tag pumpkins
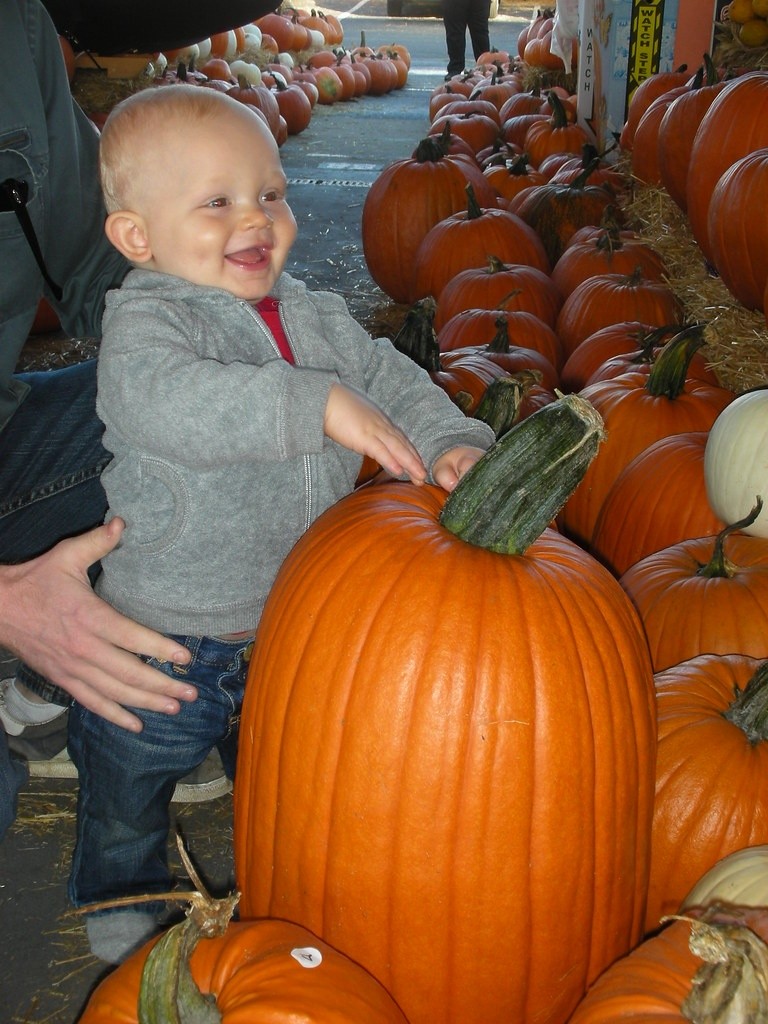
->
[53,834,410,1024]
[55,6,412,147]
[563,907,768,1024]
[229,386,655,1024]
[361,0,768,935]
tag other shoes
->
[444,73,459,81]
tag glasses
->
[1,178,65,302]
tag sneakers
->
[0,678,233,804]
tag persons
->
[63,84,500,968]
[442,0,493,81]
[0,0,234,846]
